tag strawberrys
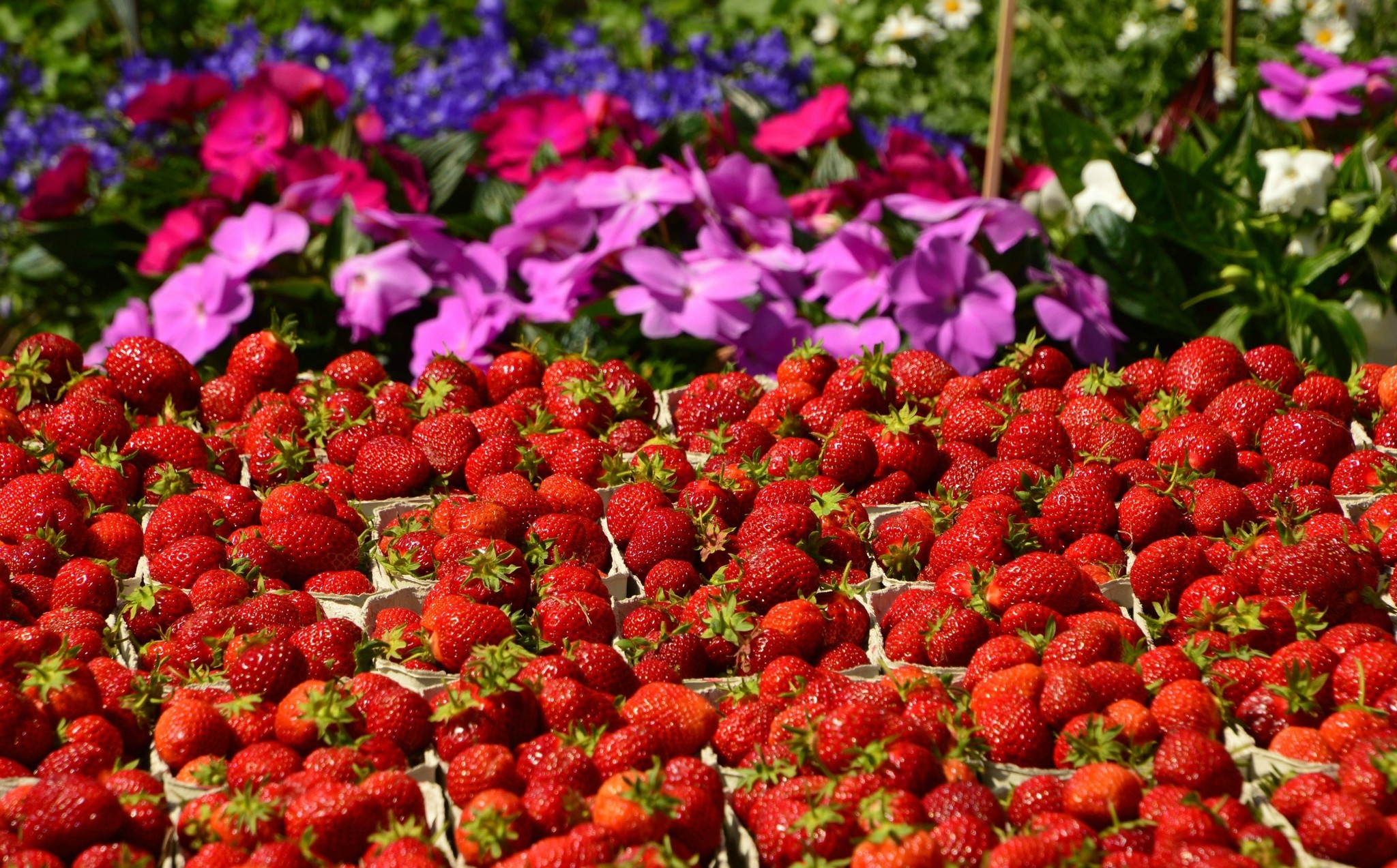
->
[0,311,1396,868]
